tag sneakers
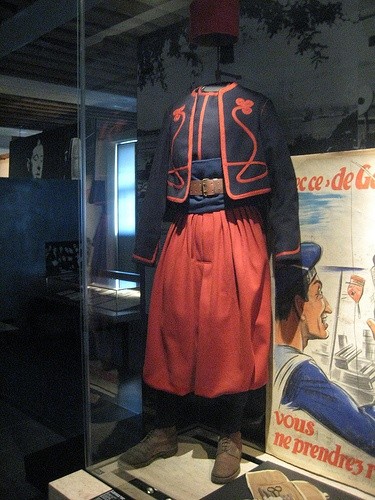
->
[211,430,242,484]
[118,427,178,470]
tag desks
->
[28,291,144,402]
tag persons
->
[117,81,301,484]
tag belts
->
[189,178,224,197]
